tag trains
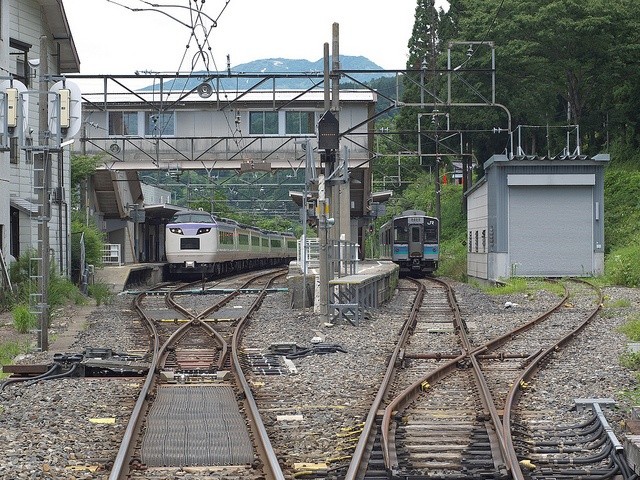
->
[376,209,440,277]
[163,207,297,282]
